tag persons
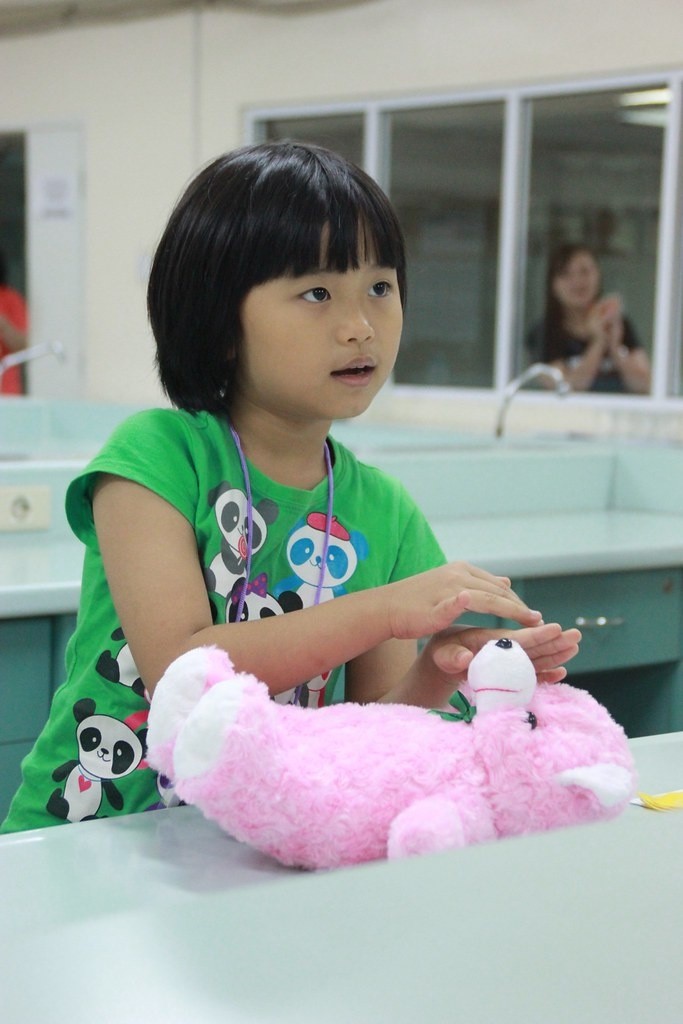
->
[525,244,651,399]
[0,145,580,837]
[0,286,29,397]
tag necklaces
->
[228,423,333,625]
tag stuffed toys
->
[144,636,637,873]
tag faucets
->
[488,362,571,434]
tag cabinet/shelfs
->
[0,509,683,832]
